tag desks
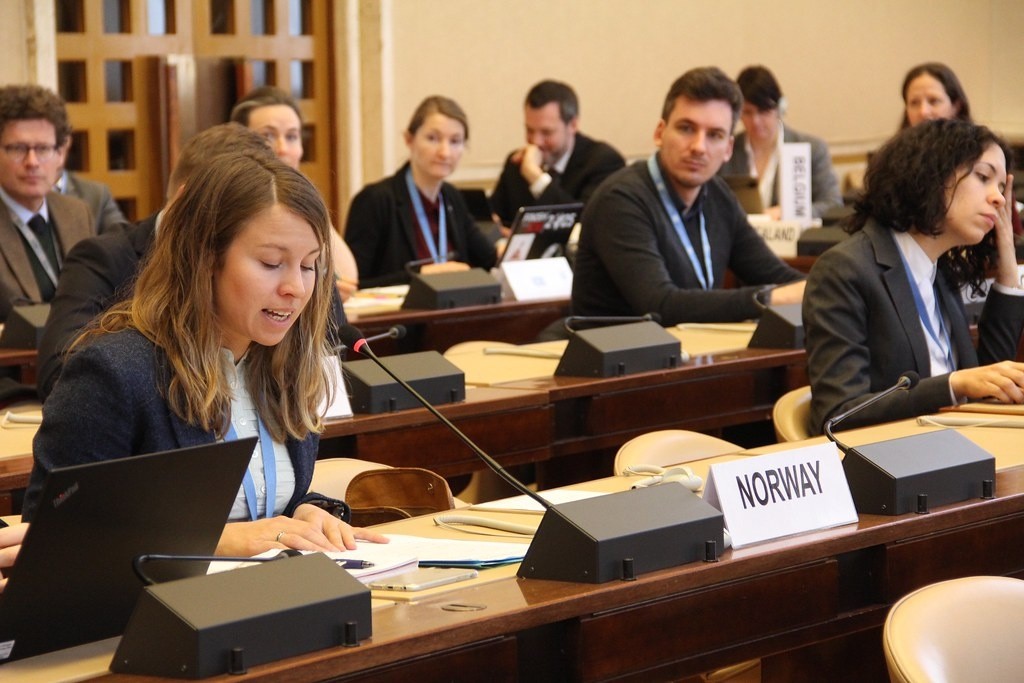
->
[0,260,1024,515]
[0,400,1024,683]
[0,252,823,388]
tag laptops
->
[496,202,587,269]
[0,436,259,664]
[719,172,764,214]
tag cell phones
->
[368,568,478,593]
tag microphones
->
[823,371,920,454]
[563,312,662,337]
[404,251,459,276]
[332,325,407,351]
[132,550,303,587]
[338,324,555,510]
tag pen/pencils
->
[332,559,374,569]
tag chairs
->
[305,339,1024,682]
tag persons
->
[892,60,1024,255]
[22,150,389,556]
[35,121,350,403]
[0,85,129,340]
[344,95,498,289]
[229,85,358,303]
[572,65,811,329]
[801,120,1024,437]
[491,80,628,258]
[720,67,843,223]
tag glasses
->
[0,142,61,161]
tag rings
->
[276,532,284,542]
